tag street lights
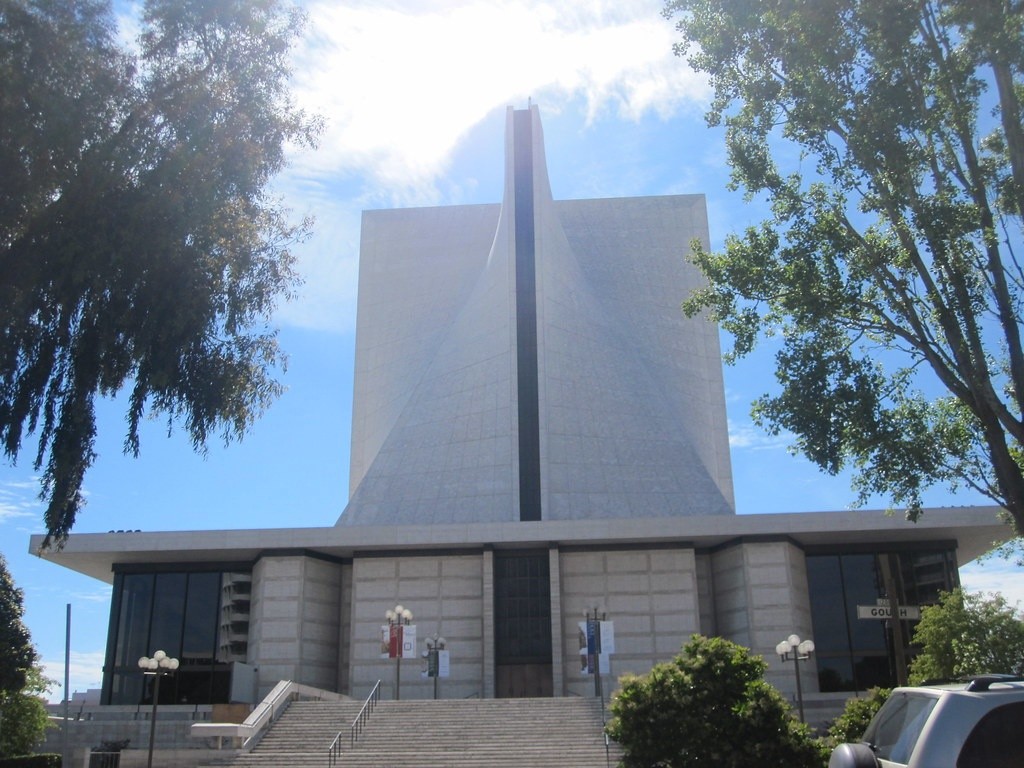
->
[775,633,814,724]
[581,599,611,698]
[138,650,180,768]
[385,605,414,700]
[425,634,447,700]
